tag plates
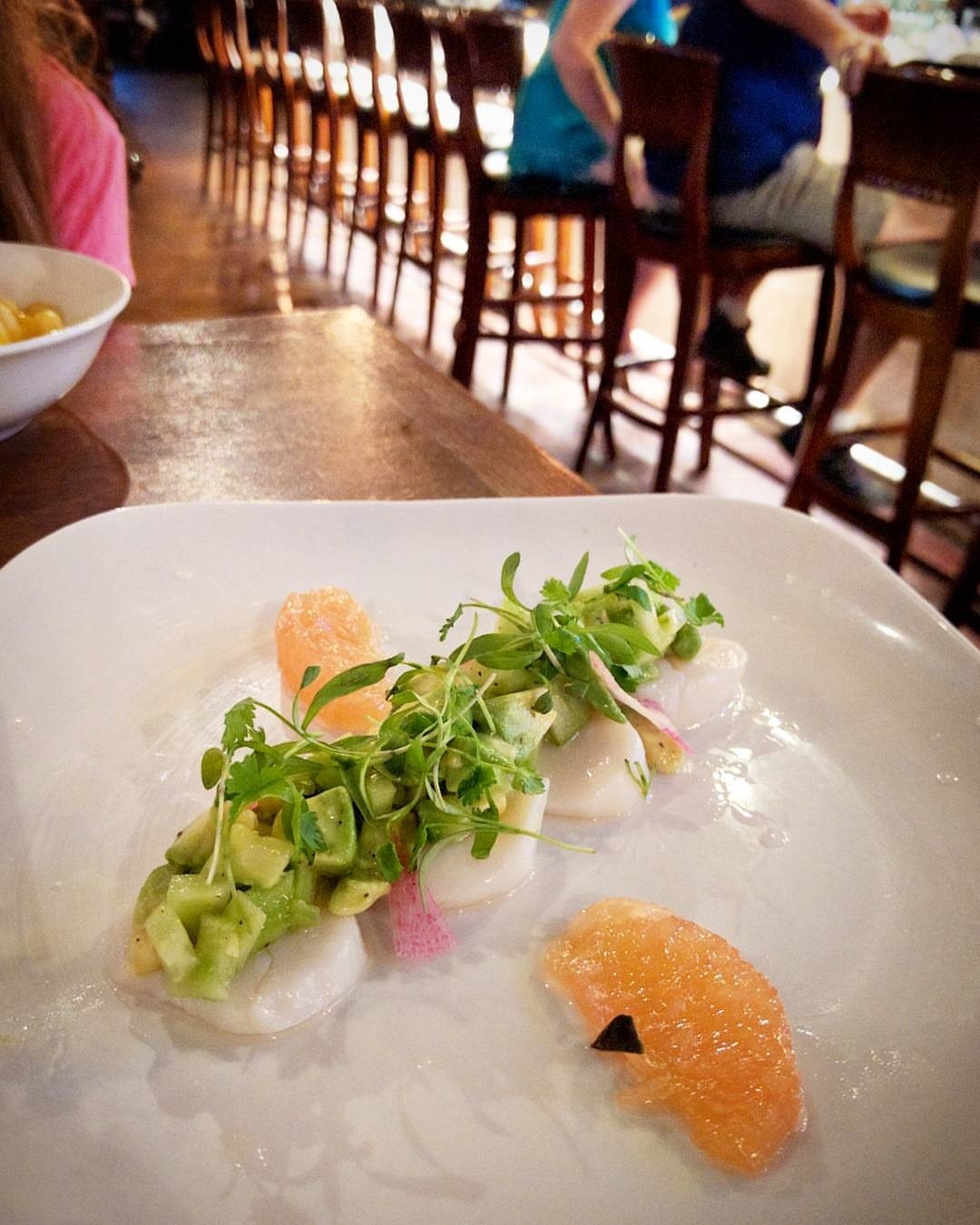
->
[0,492,980,1225]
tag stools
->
[200,0,980,637]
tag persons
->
[0,0,135,294]
[645,0,940,507]
[508,0,678,369]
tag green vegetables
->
[201,549,730,884]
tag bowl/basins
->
[1,240,133,443]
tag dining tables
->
[0,305,598,566]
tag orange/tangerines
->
[553,903,805,1174]
[277,587,394,734]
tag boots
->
[707,316,772,378]
